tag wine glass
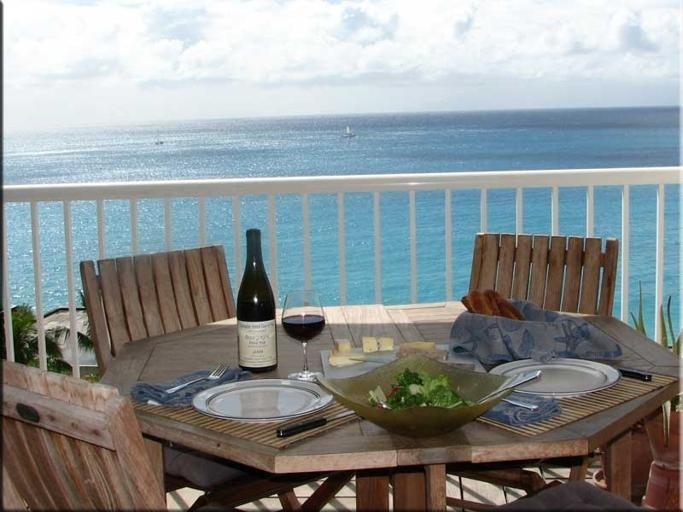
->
[281,289,325,382]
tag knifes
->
[277,408,356,438]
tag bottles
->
[236,229,278,374]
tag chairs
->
[1,235,680,512]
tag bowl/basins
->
[317,351,524,437]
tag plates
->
[486,358,621,398]
[191,379,334,420]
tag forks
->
[165,364,227,394]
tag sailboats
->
[342,125,357,139]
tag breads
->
[330,337,440,365]
[459,288,523,321]
